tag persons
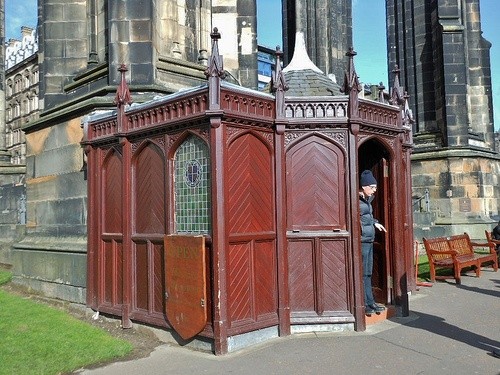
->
[356,170,388,314]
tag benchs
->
[422,232,498,287]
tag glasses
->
[369,185,377,190]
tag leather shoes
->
[365,305,374,314]
[370,303,385,312]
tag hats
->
[361,170,377,186]
